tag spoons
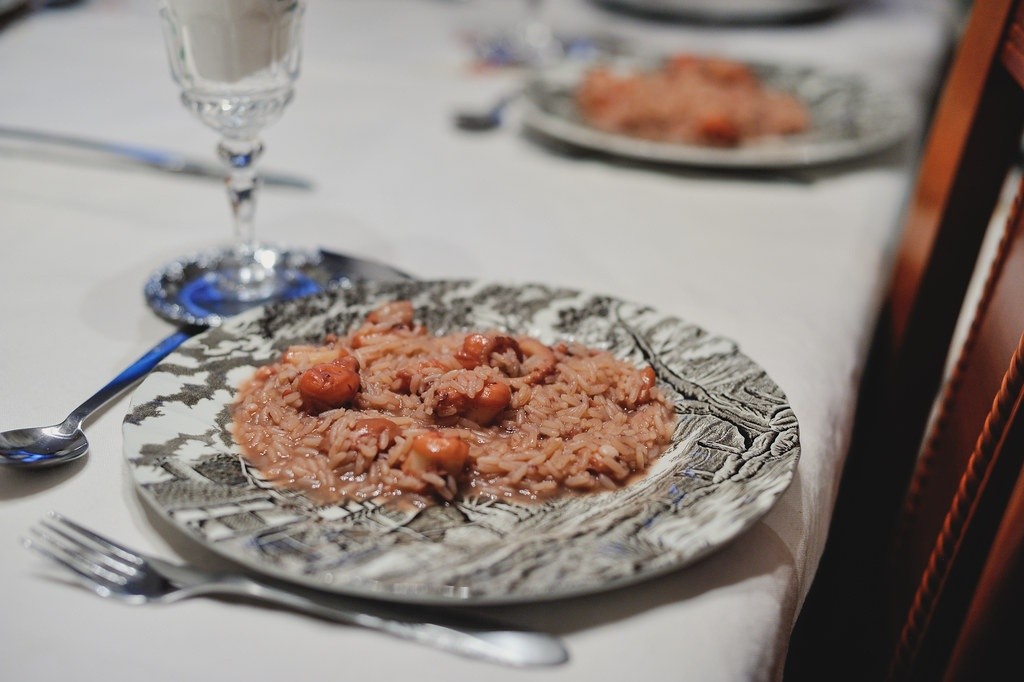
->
[0,323,206,470]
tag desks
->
[0,0,968,682]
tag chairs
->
[872,0,1024,682]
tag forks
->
[22,513,568,665]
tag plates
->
[522,41,924,166]
[122,278,801,605]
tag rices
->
[231,313,673,508]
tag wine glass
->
[145,0,368,325]
